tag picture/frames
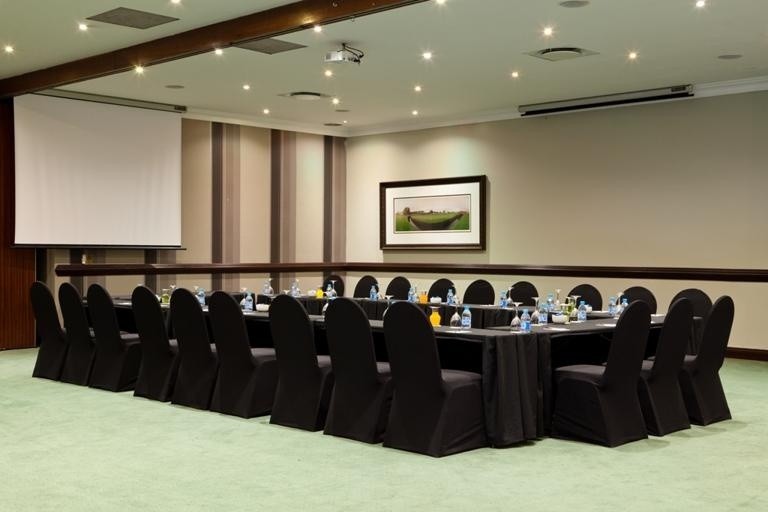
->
[379,174,486,252]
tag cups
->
[161,289,170,305]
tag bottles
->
[238,286,253,312]
[323,283,337,299]
[607,291,626,317]
[500,287,514,307]
[428,306,442,327]
[537,304,548,325]
[407,284,419,303]
[509,301,522,327]
[461,304,471,330]
[290,283,302,297]
[369,284,379,301]
[520,309,532,334]
[419,290,429,304]
[262,283,274,297]
[546,287,593,325]
[447,284,461,305]
[193,283,206,307]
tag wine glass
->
[531,296,539,325]
[382,293,394,319]
[448,301,461,330]
